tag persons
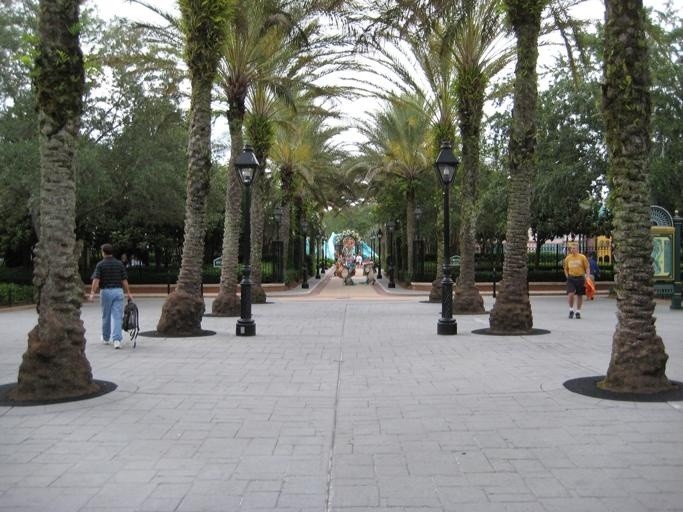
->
[89,242,134,350]
[562,242,590,320]
[585,251,600,301]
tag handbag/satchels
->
[123,298,139,348]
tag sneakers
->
[114,340,121,349]
[102,336,110,346]
[569,312,574,318]
[576,313,580,318]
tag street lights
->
[435,137,459,335]
[386,221,395,288]
[368,230,376,265]
[413,206,421,275]
[301,220,308,289]
[232,142,260,337]
[377,228,382,279]
[316,229,327,280]
[271,205,281,284]
